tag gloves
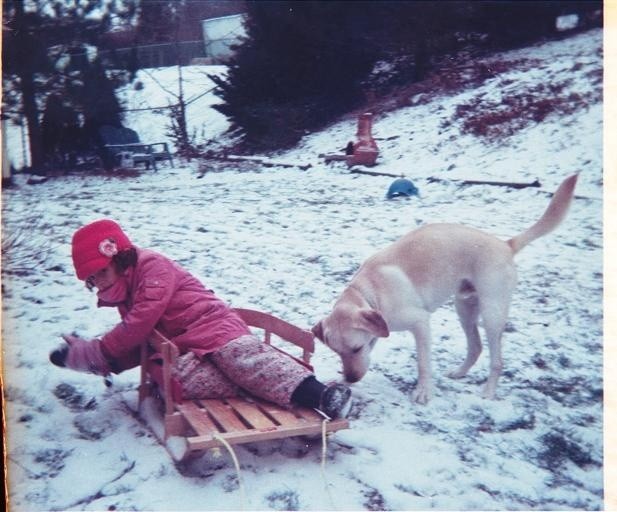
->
[51,331,112,381]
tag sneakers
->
[320,383,352,420]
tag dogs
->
[311,173,578,406]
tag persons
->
[50,219,352,422]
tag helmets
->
[387,179,418,199]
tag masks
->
[97,277,128,303]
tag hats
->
[72,219,132,279]
